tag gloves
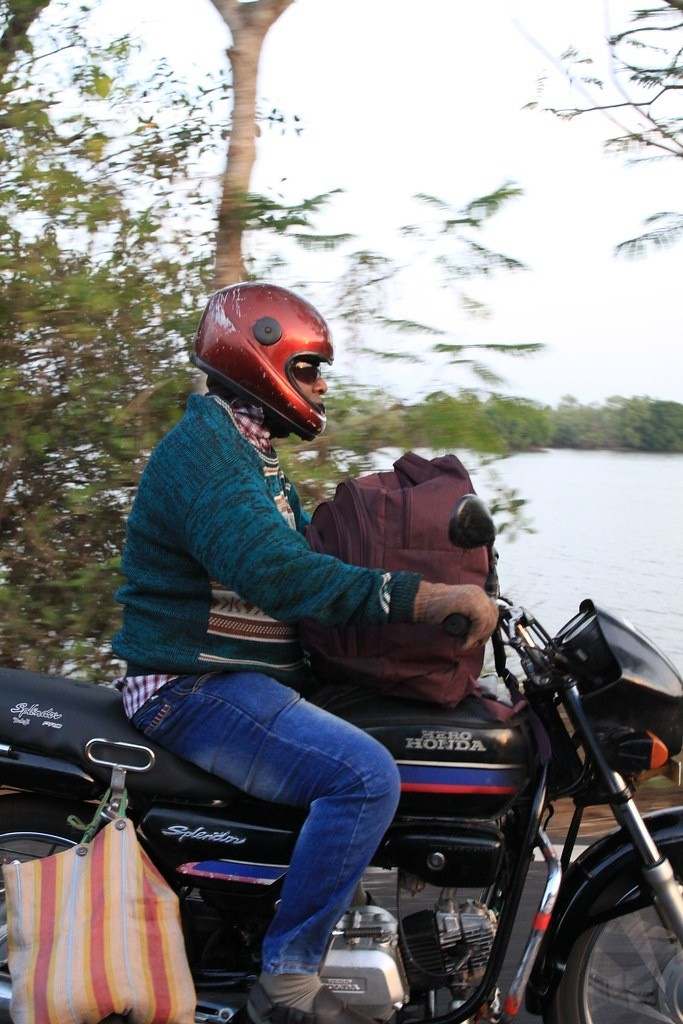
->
[413,580,500,650]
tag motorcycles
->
[1,493,683,1024]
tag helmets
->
[189,282,334,441]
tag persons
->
[114,284,498,1024]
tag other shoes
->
[247,980,380,1024]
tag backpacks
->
[304,450,552,764]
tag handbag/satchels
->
[2,788,197,1024]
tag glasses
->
[291,360,321,384]
